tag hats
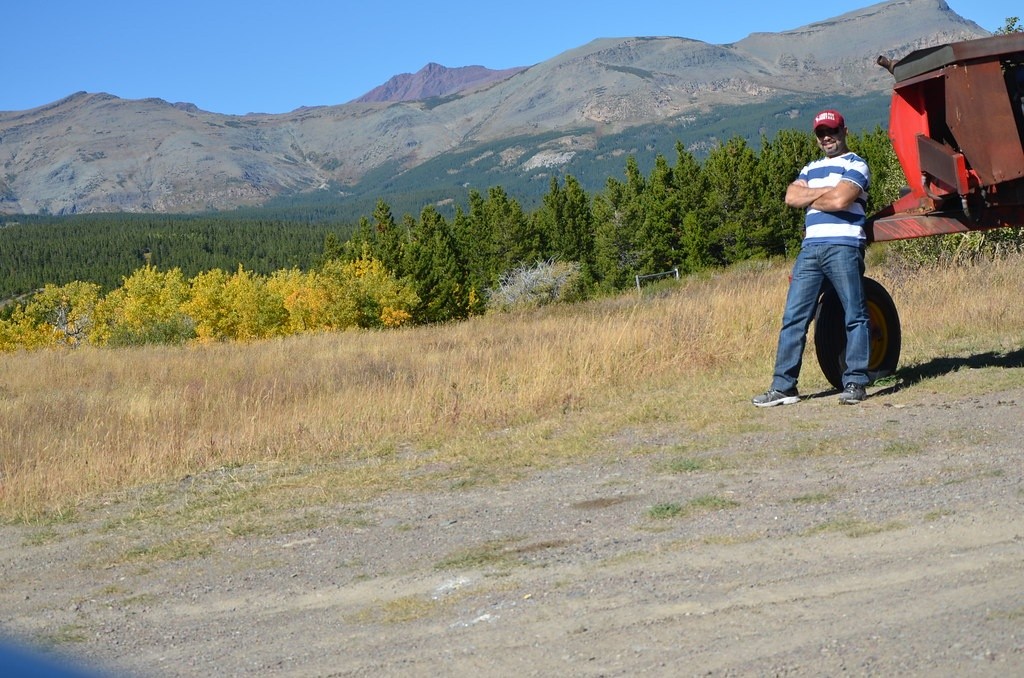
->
[813,111,844,132]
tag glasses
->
[816,128,839,137]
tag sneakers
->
[839,383,867,404]
[752,387,801,407]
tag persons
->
[750,109,873,408]
